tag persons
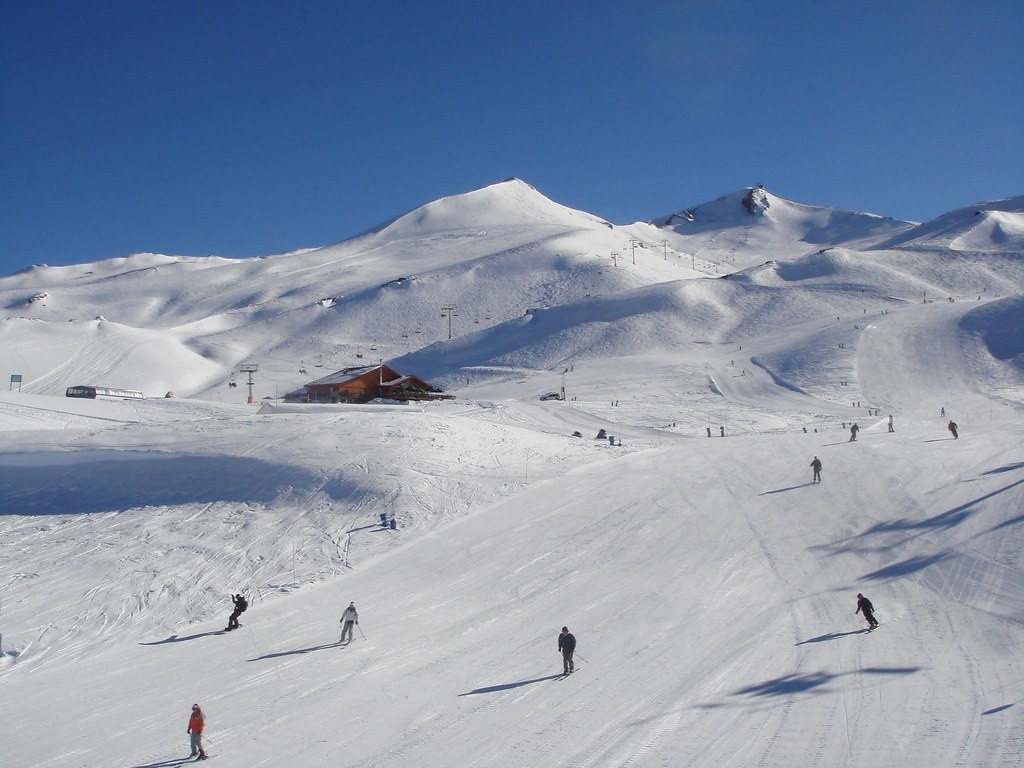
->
[187,704,206,759]
[855,593,879,629]
[558,626,577,673]
[225,594,244,631]
[850,423,860,442]
[810,456,822,484]
[948,420,958,439]
[338,601,359,643]
[888,415,895,432]
[940,407,946,418]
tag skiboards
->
[333,637,357,650]
[860,624,883,632]
[553,667,580,682]
[167,752,219,768]
[813,480,820,486]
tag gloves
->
[187,731,190,734]
[340,620,342,622]
[232,595,234,598]
[356,621,358,625]
[559,649,561,652]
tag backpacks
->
[240,601,248,611]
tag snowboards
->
[220,623,243,634]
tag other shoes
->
[190,752,198,756]
[564,670,568,674]
[570,669,573,673]
[232,625,238,628]
[870,624,874,629]
[875,624,879,628]
[225,628,231,630]
[348,640,351,643]
[198,753,206,758]
[340,639,344,642]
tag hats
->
[236,594,240,598]
[562,626,568,631]
[351,602,354,605]
[192,703,199,709]
[857,593,863,598]
